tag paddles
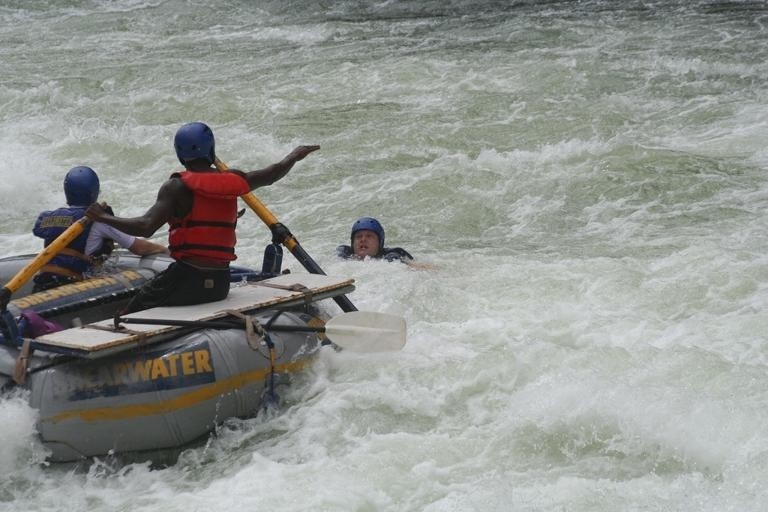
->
[111,309,407,355]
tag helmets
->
[62,165,99,205]
[175,122,216,169]
[350,217,384,257]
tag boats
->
[0,252,334,472]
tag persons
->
[338,217,414,262]
[84,122,321,314]
[32,165,171,292]
